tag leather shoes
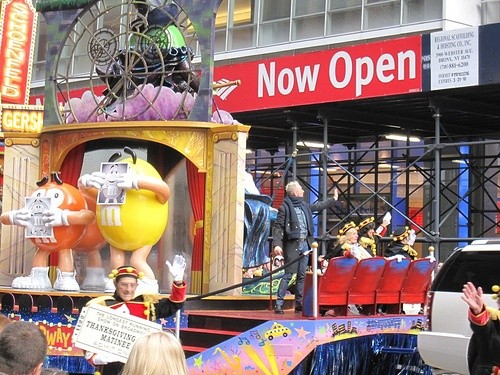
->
[275,304,284,314]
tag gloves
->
[407,234,417,246]
[383,212,392,226]
[166,254,186,284]
[350,243,359,256]
[93,353,118,365]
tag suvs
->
[417,239,500,375]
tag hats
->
[108,266,144,280]
[390,226,411,241]
[356,217,375,231]
[337,221,356,238]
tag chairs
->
[303,257,436,317]
[372,335,420,375]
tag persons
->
[243,255,287,278]
[81,254,189,375]
[273,181,339,314]
[460,282,500,375]
[333,212,418,264]
[0,322,48,375]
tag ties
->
[295,307,303,313]
